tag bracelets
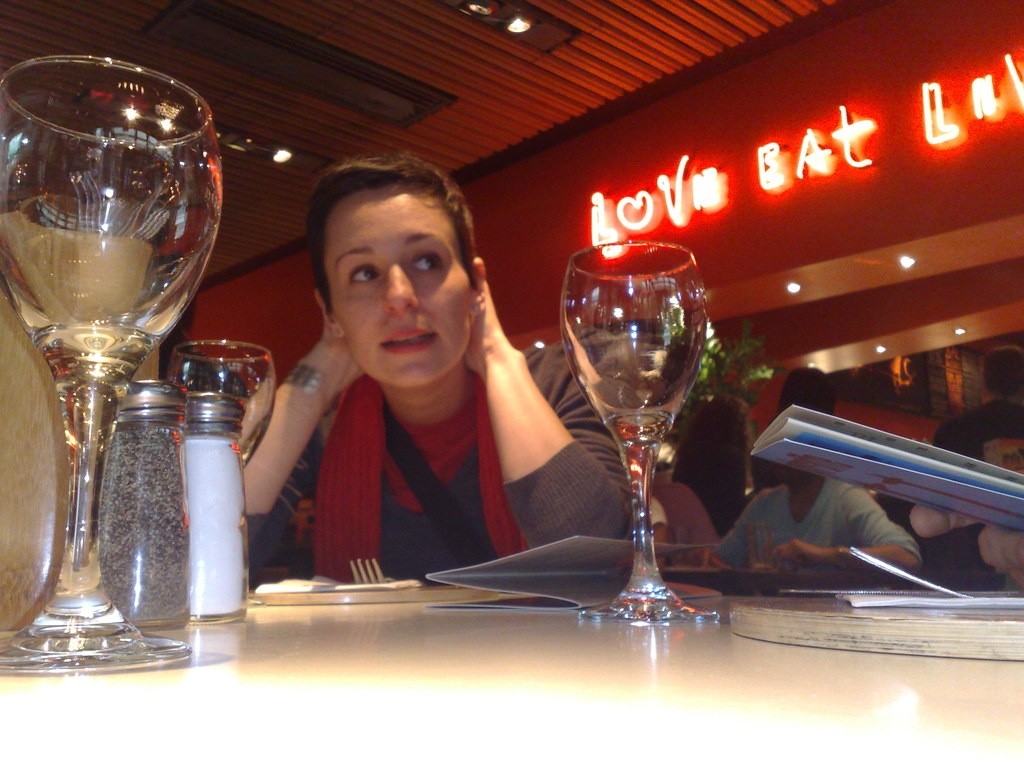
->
[285,362,341,408]
[838,546,847,566]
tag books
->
[730,602,1024,661]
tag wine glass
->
[0,57,221,670]
[168,340,275,605]
[561,243,721,622]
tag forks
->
[350,556,384,585]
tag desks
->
[662,566,862,596]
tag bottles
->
[184,391,249,616]
[91,379,194,628]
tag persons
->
[243,151,633,586]
[648,345,1024,587]
